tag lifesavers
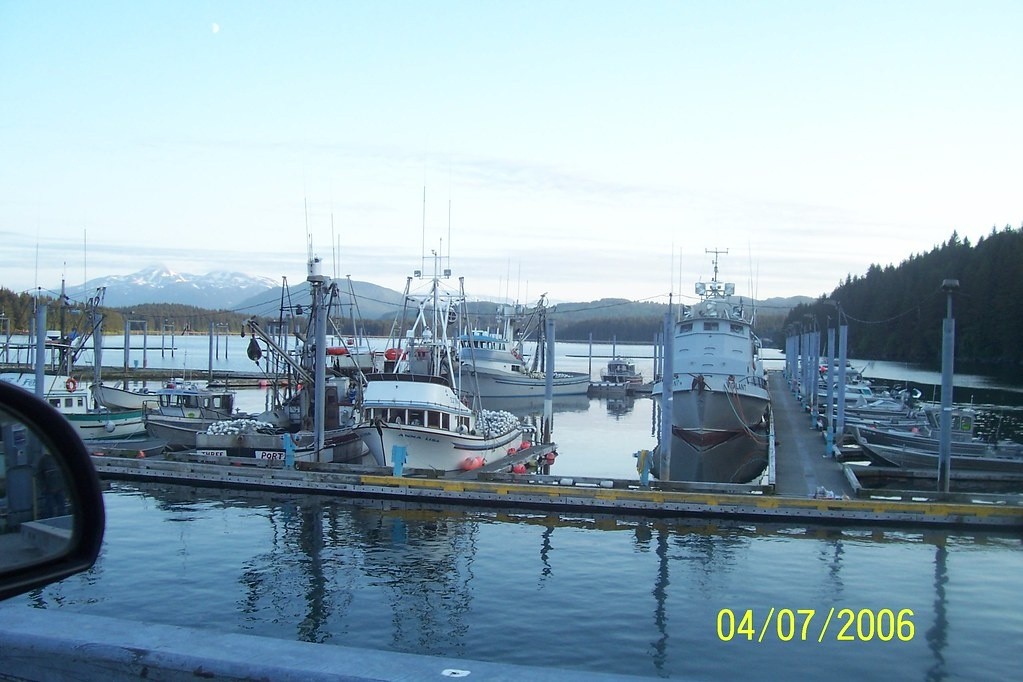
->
[66,378,77,392]
[385,348,406,361]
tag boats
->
[787,358,1023,487]
[0,189,593,469]
[599,355,643,385]
[653,246,769,429]
[644,421,771,486]
